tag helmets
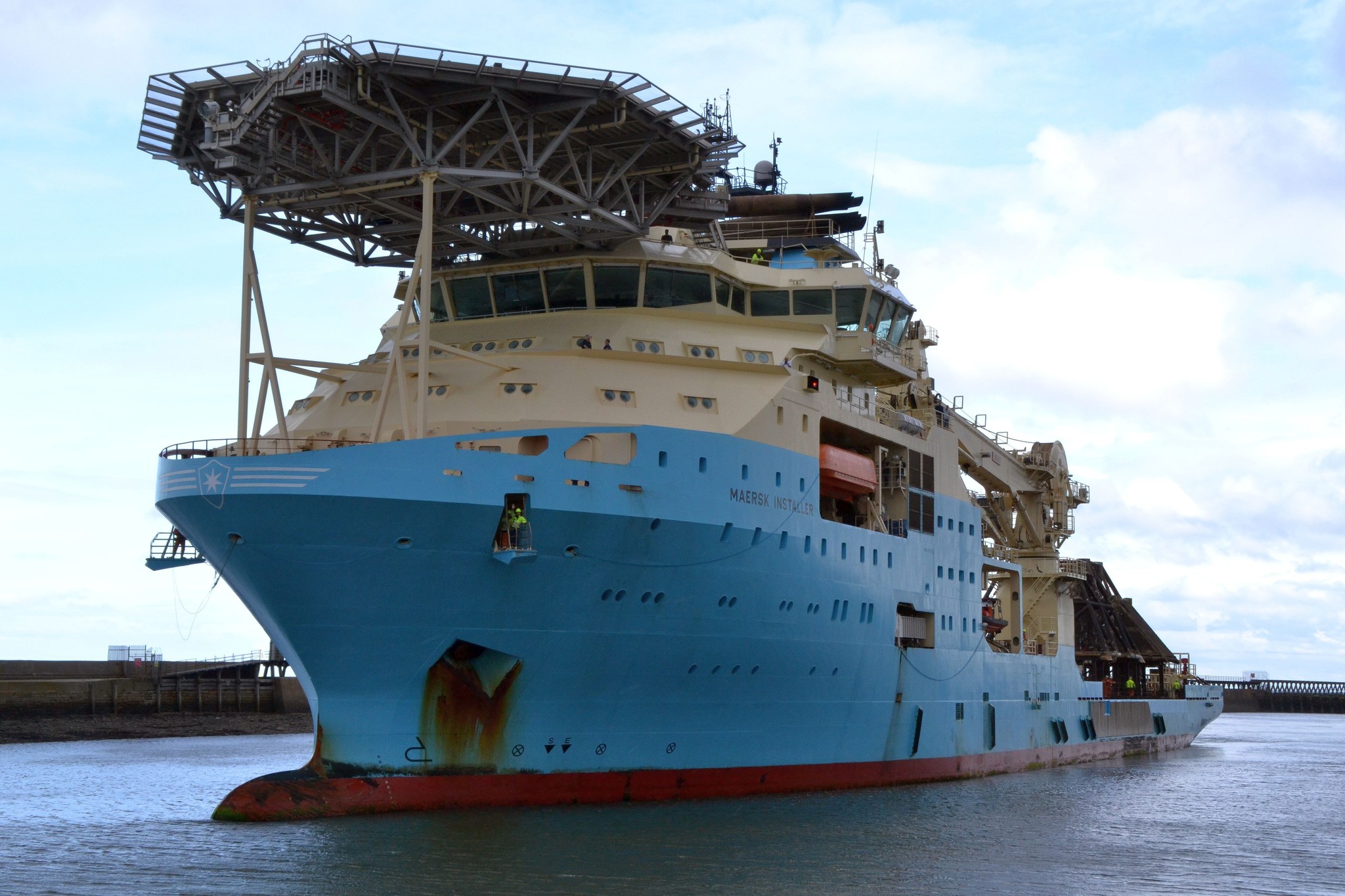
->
[507,510,511,514]
[516,508,521,512]
[1129,677,1132,679]
[1109,676,1113,678]
[1105,675,1109,677]
[1176,680,1178,682]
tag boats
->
[137,31,1231,819]
[814,443,885,512]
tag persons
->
[872,339,874,345]
[1023,629,1028,641]
[1174,679,1181,699]
[1183,663,1188,675]
[1102,675,1116,699]
[172,528,187,560]
[661,229,673,244]
[935,401,943,428]
[1125,677,1136,698]
[896,456,906,486]
[780,356,792,368]
[869,323,873,332]
[581,335,592,349]
[499,504,528,549]
[882,458,890,486]
[943,405,951,429]
[751,249,763,265]
[603,339,612,350]
